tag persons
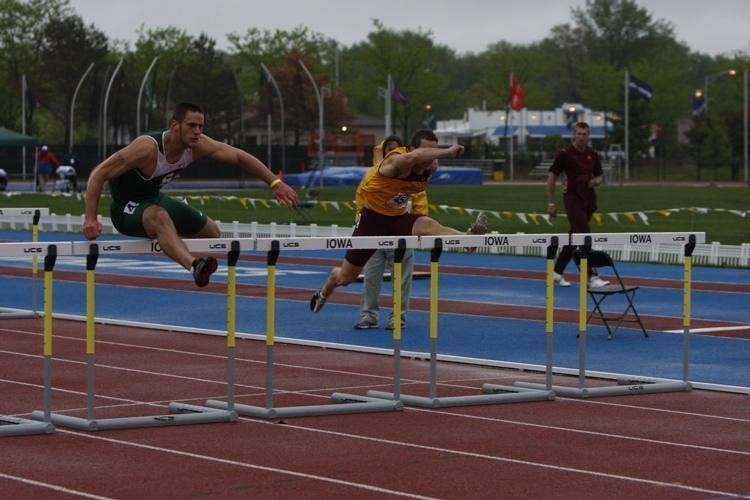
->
[36,145,59,191]
[310,129,488,313]
[83,102,299,287]
[547,122,611,288]
[61,146,81,192]
[354,136,415,329]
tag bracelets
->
[548,203,555,206]
[270,179,282,187]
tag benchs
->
[529,159,618,184]
[438,158,497,179]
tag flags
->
[629,76,654,102]
[392,80,407,104]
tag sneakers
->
[463,211,489,255]
[310,289,327,313]
[353,312,407,331]
[553,270,570,288]
[191,255,218,288]
[588,275,611,290]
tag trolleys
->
[52,163,82,195]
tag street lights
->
[704,67,737,180]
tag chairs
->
[567,249,652,340]
[288,188,322,226]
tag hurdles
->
[0,208,50,320]
[32,237,254,433]
[204,236,418,422]
[368,233,570,409]
[0,241,73,437]
[513,232,706,398]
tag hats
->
[42,145,48,151]
